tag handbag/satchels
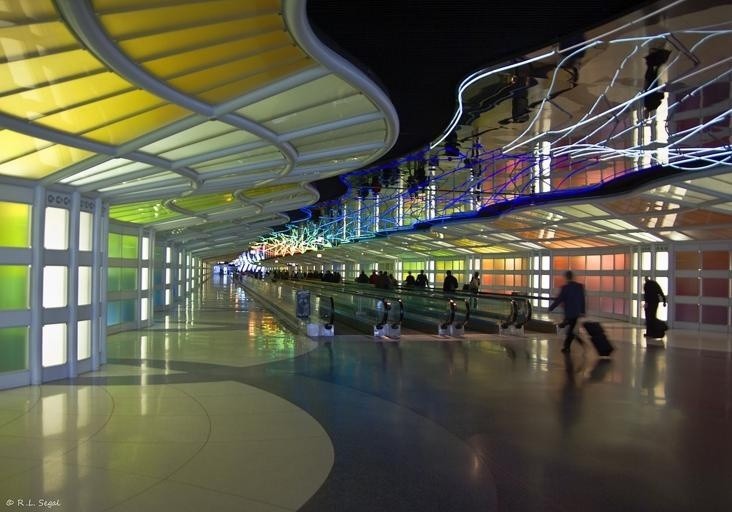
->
[583,321,604,336]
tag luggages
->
[590,335,612,356]
[647,312,667,338]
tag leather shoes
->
[561,348,569,352]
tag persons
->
[642,275,666,336]
[467,271,480,308]
[234,268,430,290]
[443,270,458,291]
[547,270,587,352]
